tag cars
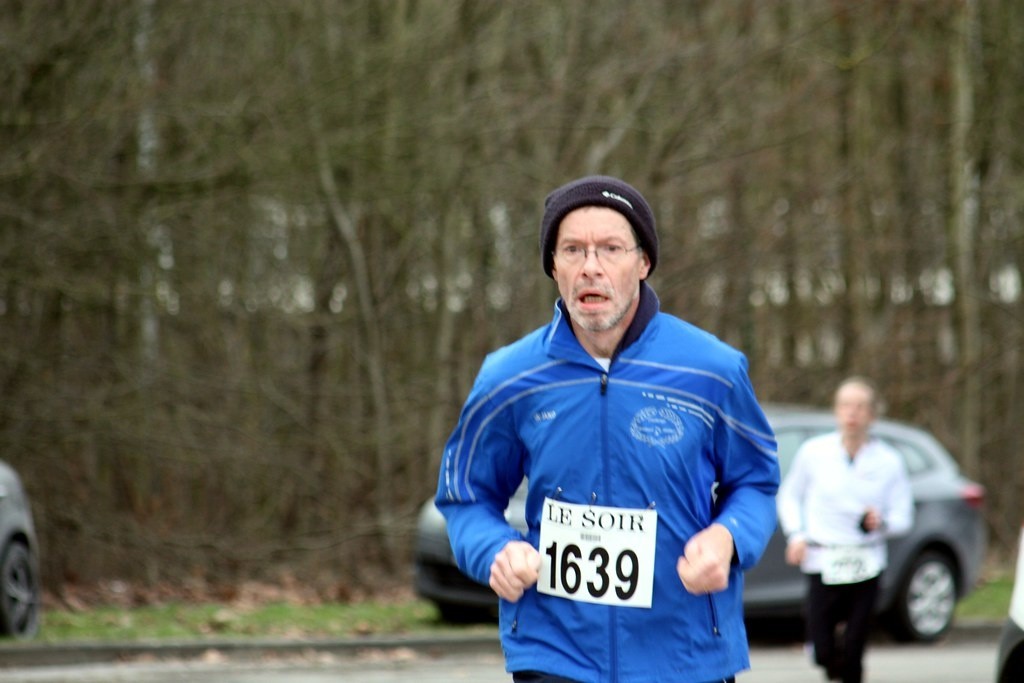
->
[0,456,48,647]
[415,403,991,645]
[988,517,1024,683]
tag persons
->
[776,376,916,683]
[435,174,780,683]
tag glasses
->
[551,244,639,264]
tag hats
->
[539,174,659,276]
